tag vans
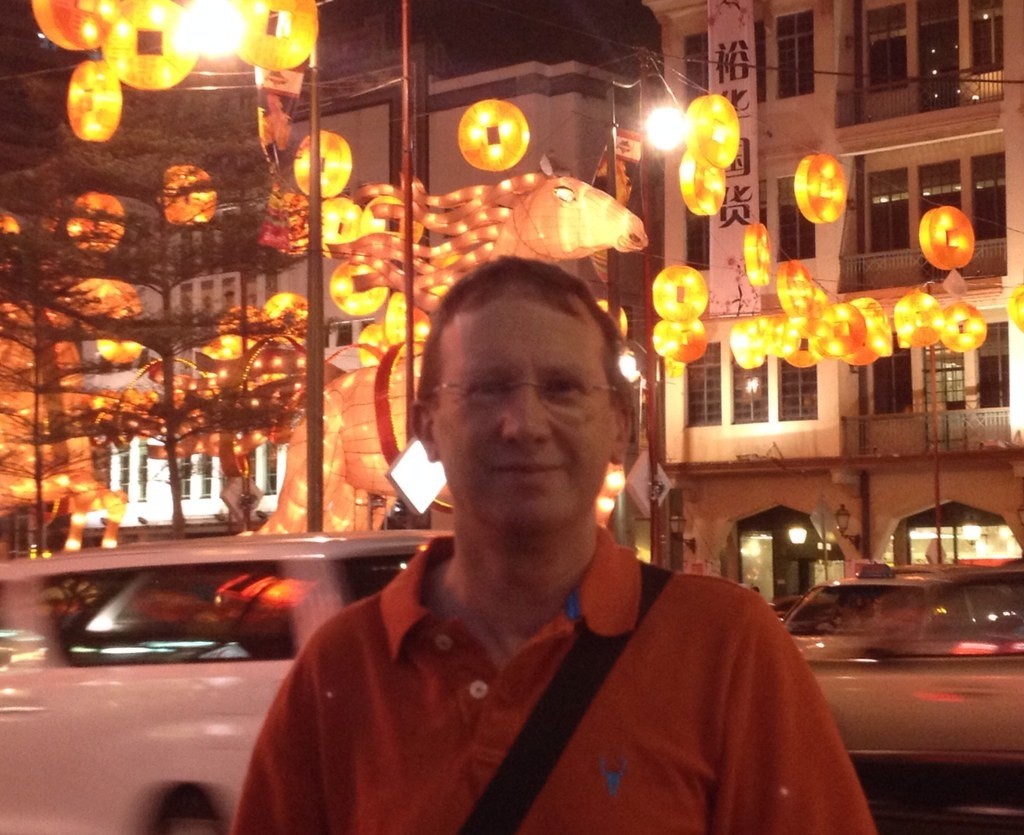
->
[1,527,454,835]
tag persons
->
[229,255,885,835]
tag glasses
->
[431,372,617,418]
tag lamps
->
[834,503,860,551]
[256,510,268,519]
[212,513,226,520]
[137,517,148,525]
[99,517,119,527]
[670,511,697,553]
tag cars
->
[786,564,1024,775]
